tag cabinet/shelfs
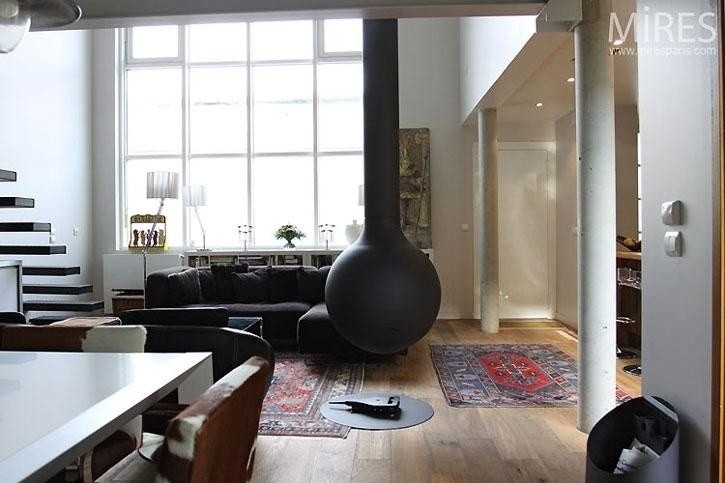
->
[183,248,434,270]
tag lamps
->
[143,171,179,253]
[183,184,211,252]
[0,0,83,53]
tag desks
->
[102,252,184,315]
[0,351,214,483]
[29,315,71,325]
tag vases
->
[284,240,295,249]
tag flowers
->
[275,225,306,240]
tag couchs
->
[145,264,346,354]
[120,307,274,384]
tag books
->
[241,256,268,267]
[210,255,237,266]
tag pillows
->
[166,264,330,308]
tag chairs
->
[0,324,146,483]
[96,356,275,483]
[0,312,28,324]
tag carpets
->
[428,342,633,407]
[258,354,366,437]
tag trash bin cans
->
[585,395,681,483]
